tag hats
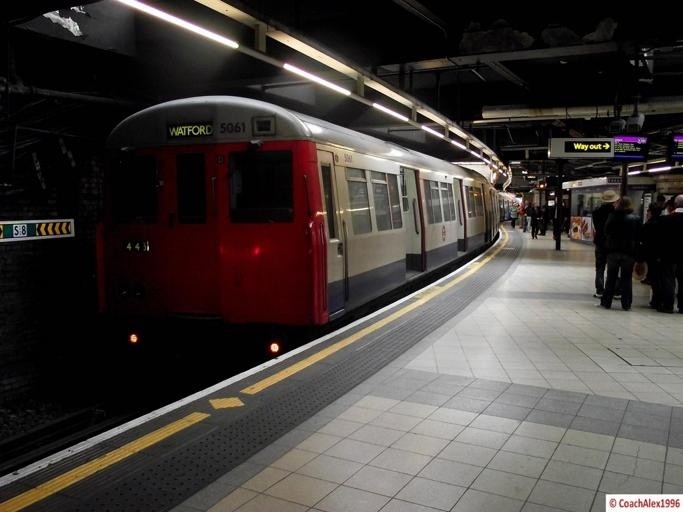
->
[599,189,620,204]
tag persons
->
[508,193,583,251]
[590,188,683,314]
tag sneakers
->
[592,289,683,314]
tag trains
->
[97,94,520,401]
